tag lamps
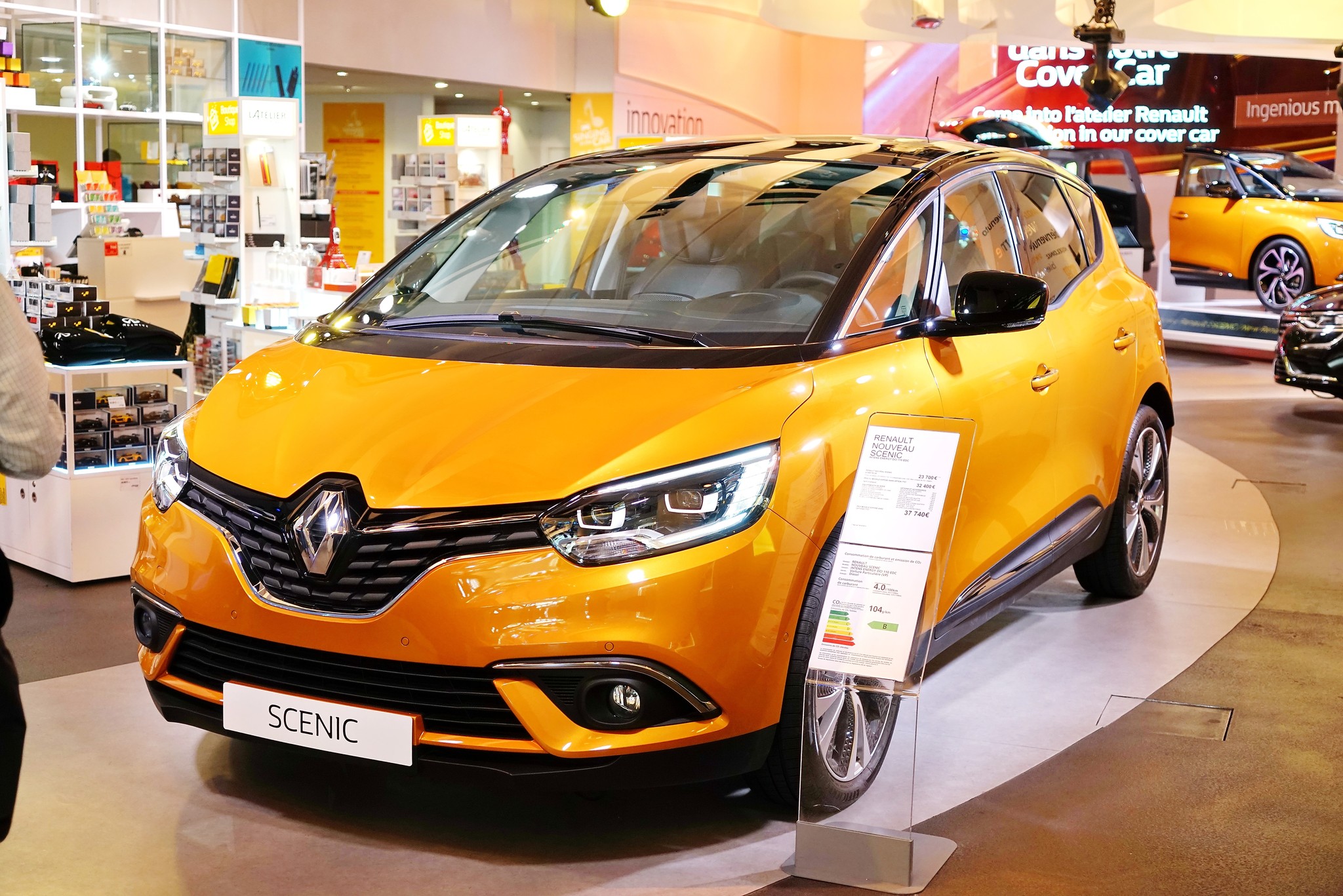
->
[1074,0,1130,113]
[587,0,630,18]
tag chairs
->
[1190,168,1229,197]
[626,187,778,302]
[761,232,826,288]
[1253,168,1286,197]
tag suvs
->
[127,118,1181,828]
[1168,141,1343,311]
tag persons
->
[1,269,68,851]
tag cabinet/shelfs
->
[392,112,506,268]
[0,1,331,417]
[1,359,196,583]
